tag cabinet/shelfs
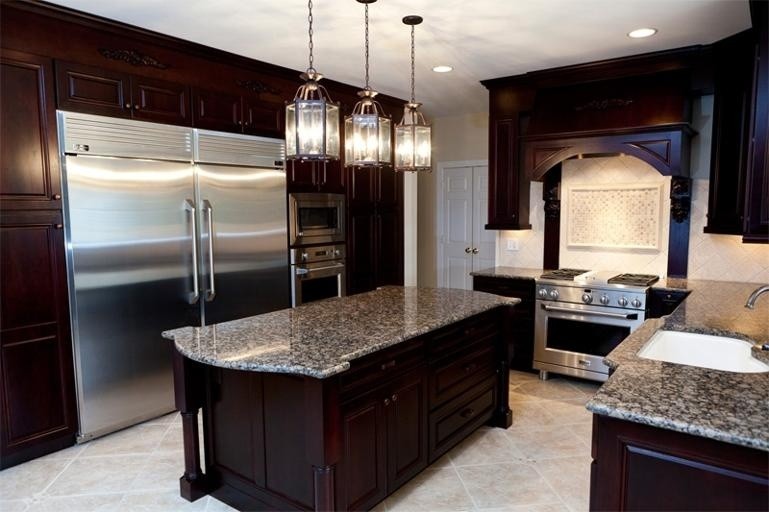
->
[342,114,406,296]
[472,273,539,374]
[285,102,347,195]
[0,44,83,474]
[50,59,193,129]
[647,289,690,319]
[703,27,768,243]
[189,84,287,140]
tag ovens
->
[531,299,647,384]
[286,191,347,309]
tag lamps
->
[341,0,392,167]
[284,0,342,164]
[393,16,434,174]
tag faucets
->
[743,285,769,309]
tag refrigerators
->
[57,108,290,447]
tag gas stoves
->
[535,268,661,311]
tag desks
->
[160,286,523,511]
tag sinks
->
[637,323,768,377]
